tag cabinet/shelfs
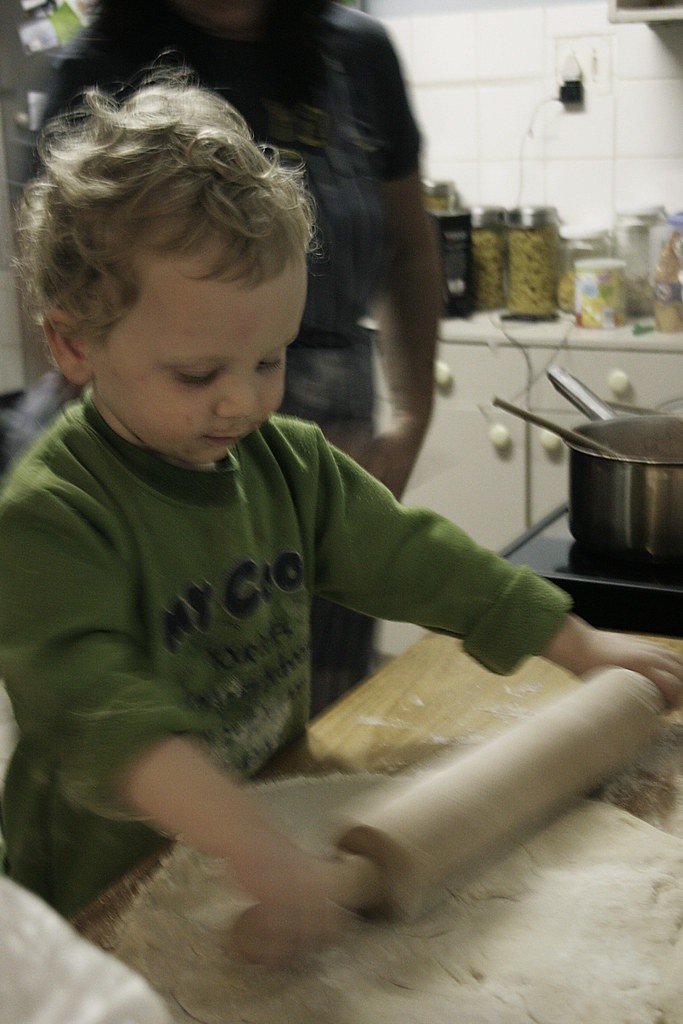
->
[372,334,683,655]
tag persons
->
[0,66,683,945]
[34,0,442,723]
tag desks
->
[70,631,683,1024]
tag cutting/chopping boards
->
[62,627,683,1024]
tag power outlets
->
[558,39,597,84]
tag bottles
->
[417,179,683,334]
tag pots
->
[546,365,683,565]
